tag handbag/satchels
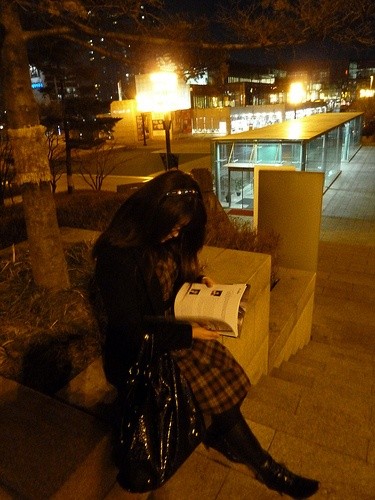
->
[114,327,205,493]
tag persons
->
[88,170,320,500]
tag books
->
[174,282,250,339]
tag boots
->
[204,424,248,466]
[224,419,320,499]
